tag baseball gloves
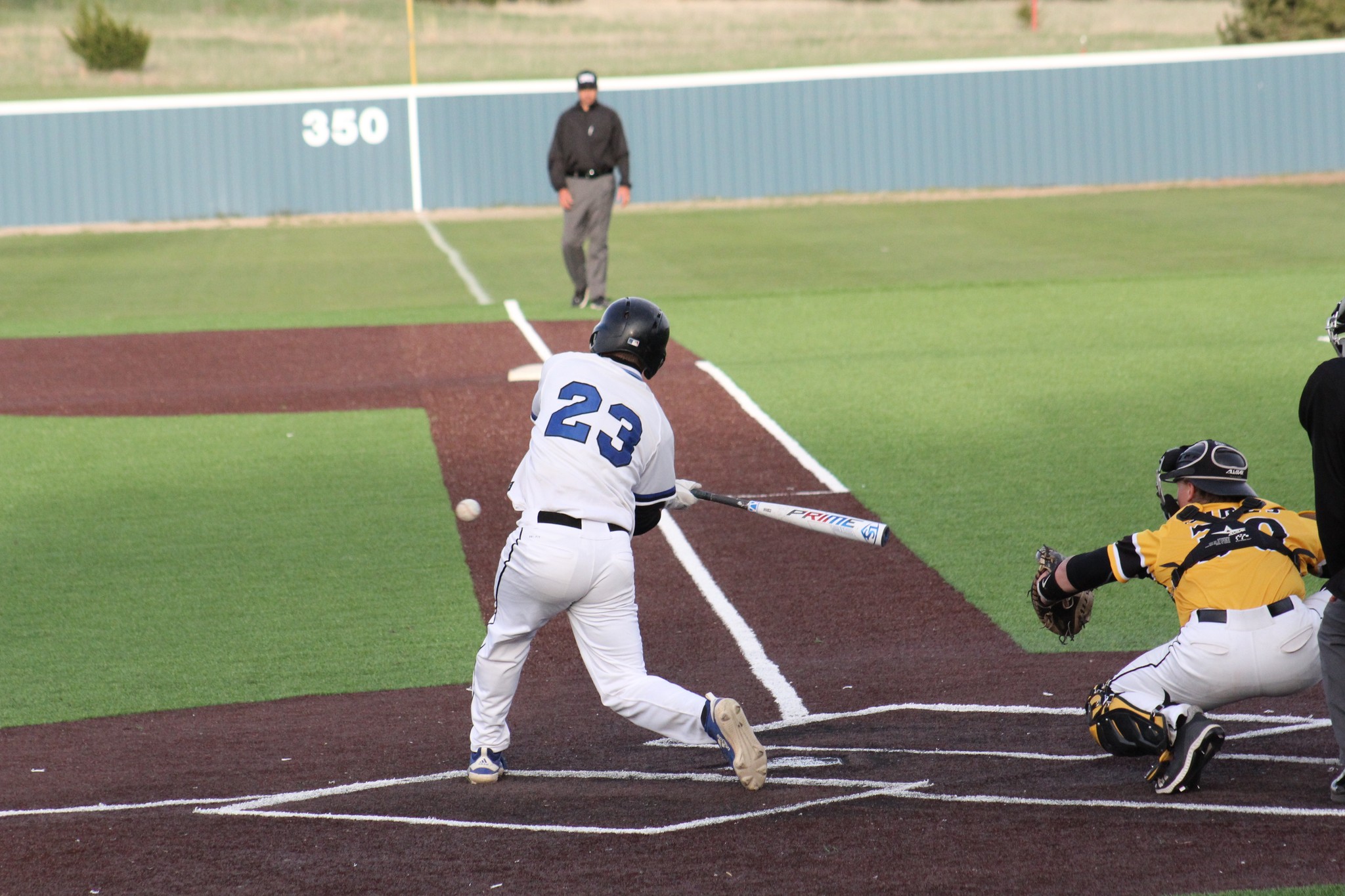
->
[1026,544,1095,637]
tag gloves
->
[666,478,702,510]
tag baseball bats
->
[690,487,892,548]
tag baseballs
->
[455,498,481,523]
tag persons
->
[548,70,632,310]
[1298,298,1345,803]
[468,297,767,790]
[1030,439,1332,794]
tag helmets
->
[1172,439,1260,498]
[589,296,670,380]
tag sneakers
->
[704,691,768,790]
[466,746,508,785]
[1154,705,1226,796]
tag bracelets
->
[1037,561,1076,603]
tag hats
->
[577,71,597,89]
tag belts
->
[536,511,631,534]
[566,167,613,178]
[1197,597,1295,623]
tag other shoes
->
[1330,767,1345,801]
[572,284,614,311]
